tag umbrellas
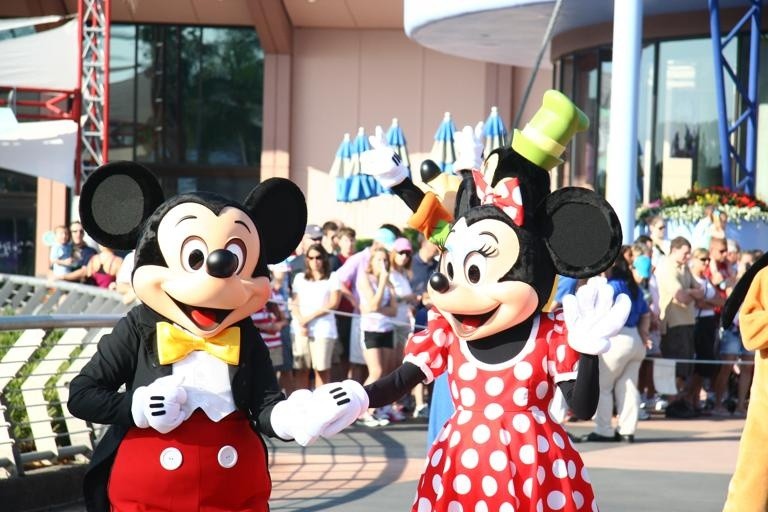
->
[331,106,506,202]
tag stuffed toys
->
[720,251,768,512]
[313,174,623,512]
[66,160,313,511]
[360,88,592,450]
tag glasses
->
[719,248,727,254]
[307,254,323,261]
[699,257,711,262]
[658,224,666,231]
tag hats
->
[305,224,324,239]
[395,237,413,254]
[373,229,396,252]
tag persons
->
[50,202,768,443]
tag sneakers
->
[638,391,747,422]
[579,432,615,442]
[354,415,391,427]
[374,402,409,422]
[413,402,429,419]
[615,430,635,443]
